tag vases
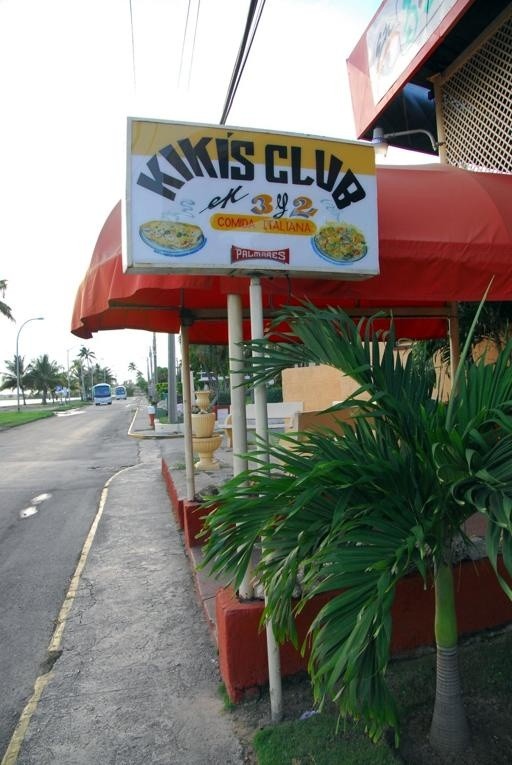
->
[195,391,211,415]
[191,435,222,471]
[191,412,216,438]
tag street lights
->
[67,345,83,406]
[17,316,44,413]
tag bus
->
[92,383,112,405]
[115,386,126,400]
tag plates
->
[309,232,367,266]
[138,227,206,257]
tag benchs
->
[219,401,304,448]
